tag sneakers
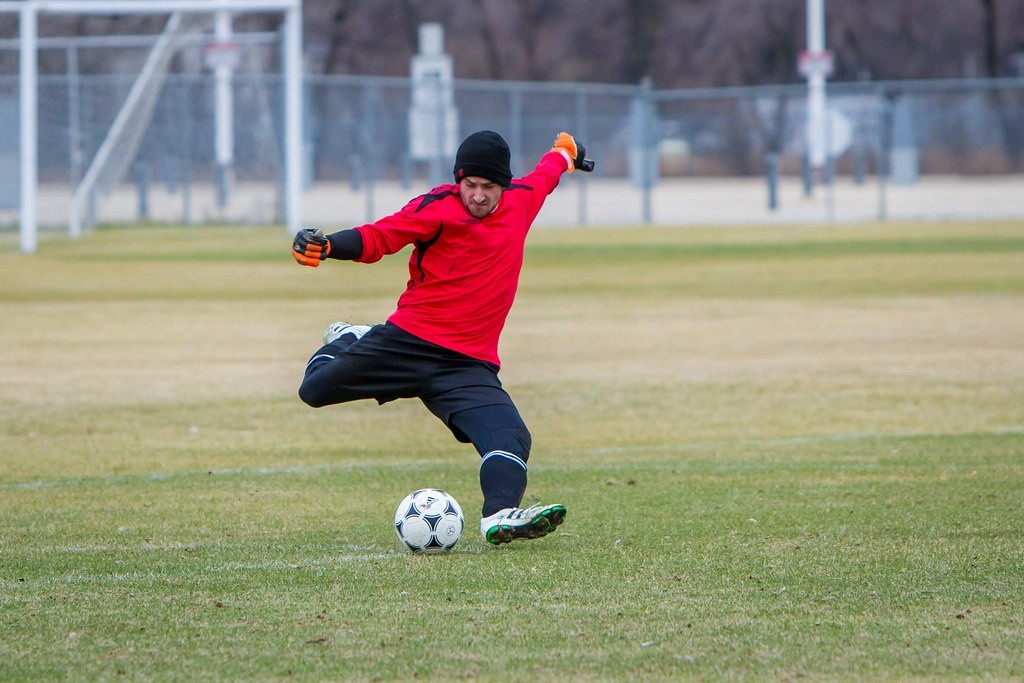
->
[480,501,568,544]
[322,321,386,338]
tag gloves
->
[291,228,363,266]
[549,131,595,171]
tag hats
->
[453,130,513,187]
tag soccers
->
[394,487,465,555]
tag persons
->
[287,131,596,546]
